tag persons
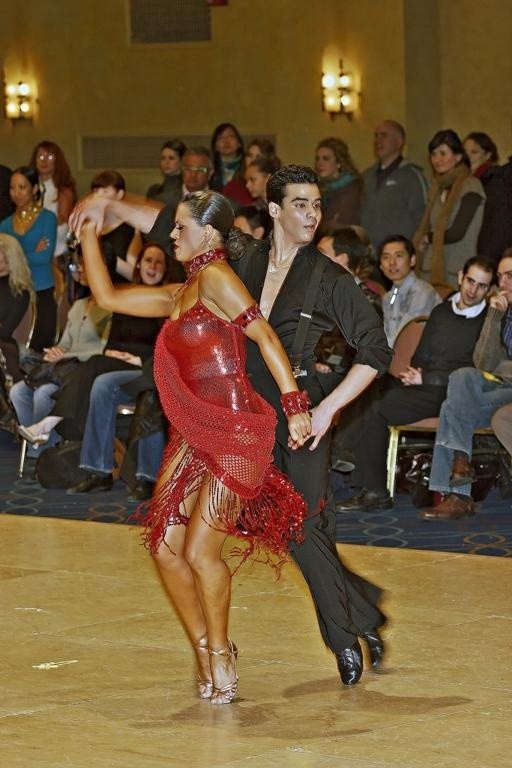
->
[0,118,511,522]
[67,164,396,685]
[77,188,310,704]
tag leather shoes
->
[66,473,114,495]
[360,624,385,667]
[336,639,363,685]
[448,458,478,486]
[335,492,392,513]
[419,494,475,521]
[328,443,356,473]
[126,480,152,503]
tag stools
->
[472,427,510,499]
[386,423,438,498]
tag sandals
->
[191,630,238,705]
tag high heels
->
[16,424,51,450]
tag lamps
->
[5,81,33,125]
[321,58,354,120]
